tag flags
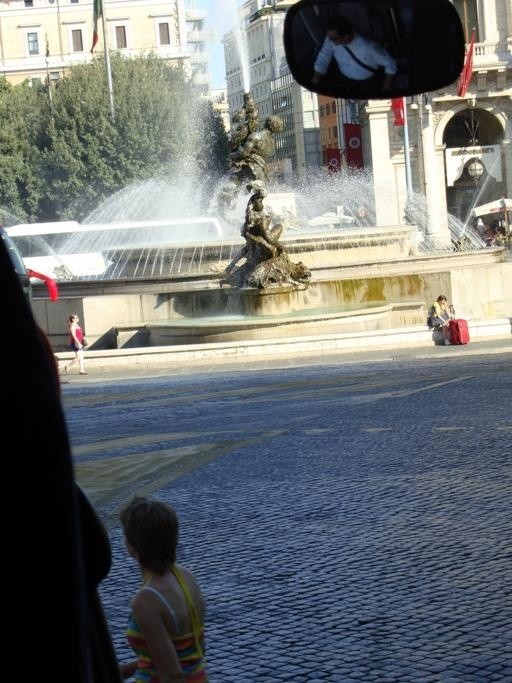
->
[90,0,103,55]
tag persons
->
[1,224,123,682]
[113,497,208,683]
[449,304,455,320]
[453,156,498,187]
[310,20,401,98]
[474,218,486,235]
[493,228,508,247]
[228,116,284,181]
[65,313,88,375]
[428,295,451,345]
[240,180,285,276]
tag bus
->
[5,217,223,282]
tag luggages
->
[448,305,469,344]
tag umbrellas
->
[468,198,512,247]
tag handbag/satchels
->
[82,338,88,346]
[427,316,436,326]
